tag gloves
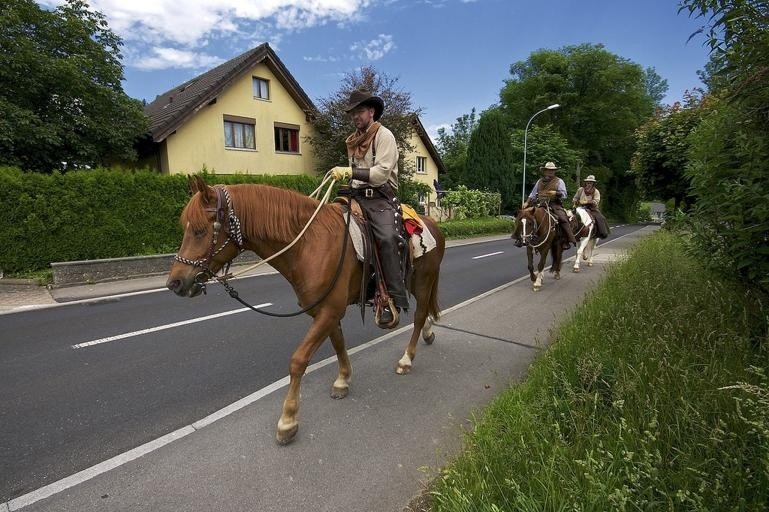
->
[329,166,353,181]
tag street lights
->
[522,102,561,210]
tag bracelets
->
[527,197,532,204]
[556,189,562,199]
[350,166,371,182]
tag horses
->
[165,172,447,447]
[565,206,601,273]
[513,205,578,293]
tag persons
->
[522,161,572,252]
[572,174,607,240]
[330,88,409,329]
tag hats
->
[540,162,559,171]
[583,175,598,182]
[344,88,384,122]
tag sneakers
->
[379,306,401,324]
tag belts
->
[357,188,381,197]
